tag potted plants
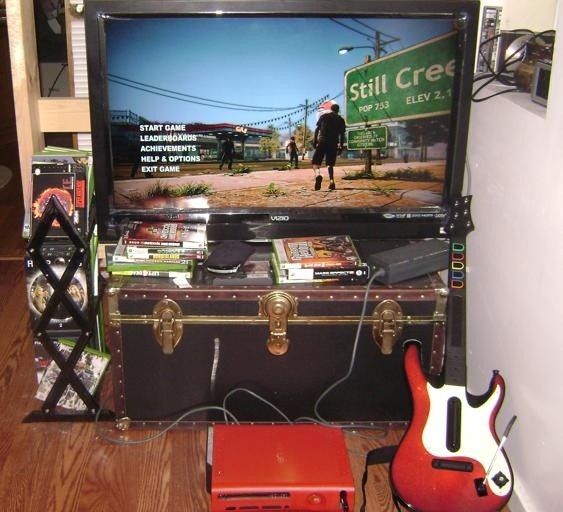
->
[126,179,213,211]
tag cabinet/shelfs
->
[15,193,116,423]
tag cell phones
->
[205,242,252,274]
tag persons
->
[218,138,236,170]
[129,121,157,179]
[312,105,346,190]
[286,137,300,169]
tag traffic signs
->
[343,124,389,151]
[344,28,457,126]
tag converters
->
[369,238,450,284]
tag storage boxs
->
[100,238,457,433]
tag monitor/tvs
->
[84,0,480,238]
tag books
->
[22,146,110,413]
[96,213,373,286]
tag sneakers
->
[315,175,322,190]
[328,183,335,190]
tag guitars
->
[389,196,514,512]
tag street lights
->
[297,122,306,161]
[337,44,387,58]
